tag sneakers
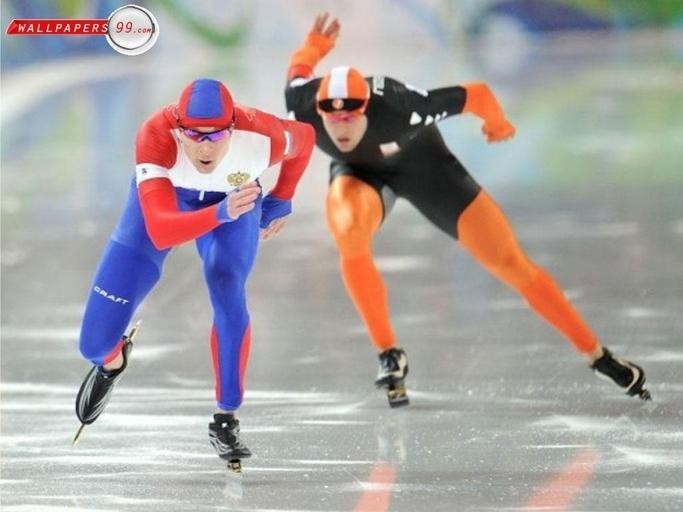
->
[208,413,252,460]
[74,333,133,425]
[373,348,410,390]
[589,345,648,397]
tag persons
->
[285,14,645,397]
[79,79,316,460]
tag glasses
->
[176,120,234,144]
[326,112,357,123]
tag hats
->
[177,78,235,129]
[315,65,371,118]
